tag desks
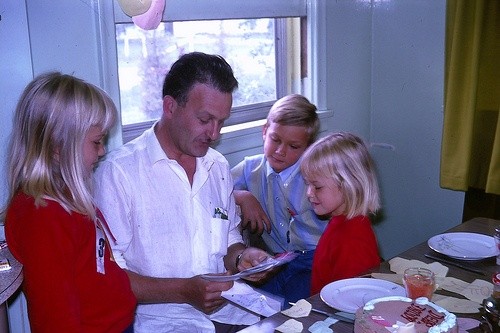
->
[235,218,500,333]
[0,239,24,333]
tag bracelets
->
[234,247,247,266]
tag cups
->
[403,267,435,302]
[493,227,500,265]
[492,272,500,303]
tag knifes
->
[287,302,355,324]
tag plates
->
[427,232,496,260]
[319,278,406,314]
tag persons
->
[90,52,280,333]
[4,74,135,333]
[304,131,380,295]
[231,95,333,311]
